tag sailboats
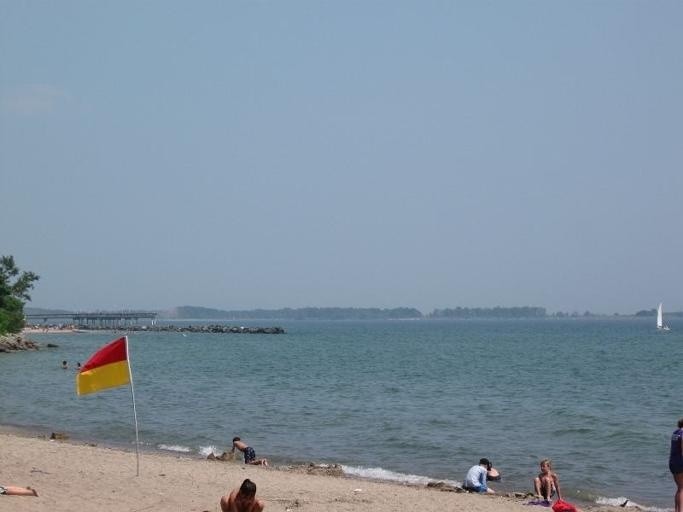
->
[653,302,671,331]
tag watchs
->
[462,457,494,494]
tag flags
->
[75,336,129,397]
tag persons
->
[668,418,683,512]
[231,437,267,467]
[220,478,263,511]
[487,464,500,482]
[0,484,39,498]
[61,361,69,369]
[77,362,81,370]
[534,459,561,502]
